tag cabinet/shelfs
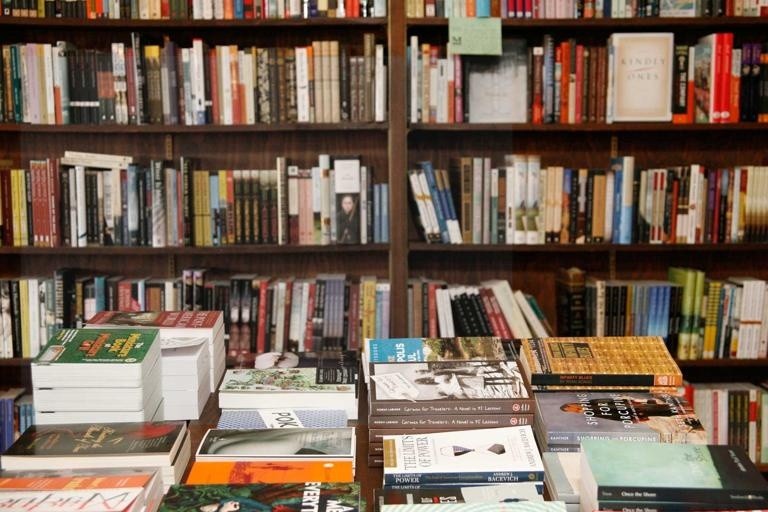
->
[1,0,768,383]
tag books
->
[0,32,388,125]
[408,268,768,359]
[408,32,768,123]
[1,309,768,512]
[1,268,390,355]
[406,0,768,18]
[0,150,389,248]
[1,0,387,20]
[409,153,768,244]
